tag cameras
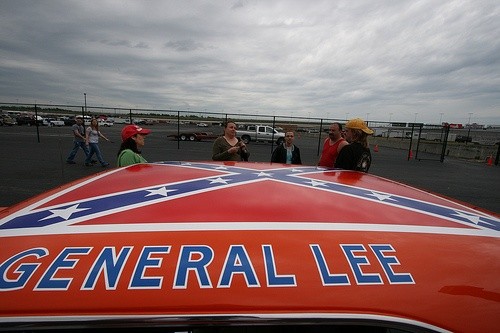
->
[237,141,250,158]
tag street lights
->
[84,92,87,111]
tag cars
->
[0,111,154,127]
[197,122,209,127]
[180,130,210,142]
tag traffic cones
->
[408,149,413,159]
[487,157,493,166]
[374,144,379,152]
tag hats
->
[122,124,152,141]
[345,118,374,134]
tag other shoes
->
[86,164,93,166]
[67,160,75,164]
[102,163,110,166]
[91,159,98,163]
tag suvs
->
[455,135,472,142]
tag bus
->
[386,122,424,138]
[449,124,483,129]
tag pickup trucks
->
[235,125,286,145]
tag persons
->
[66,118,98,164]
[211,121,250,162]
[116,124,153,167]
[316,123,351,167]
[333,119,375,173]
[84,119,112,167]
[270,131,301,165]
